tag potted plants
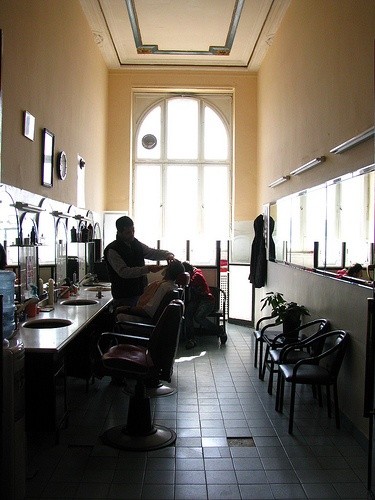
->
[259,291,309,340]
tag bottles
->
[31,226,35,245]
[82,225,89,242]
[88,224,93,242]
[0,270,16,339]
[71,226,77,242]
[79,225,83,241]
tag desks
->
[261,331,302,392]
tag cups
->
[24,298,37,317]
[24,238,29,245]
[61,285,69,299]
[16,238,21,244]
[70,285,78,296]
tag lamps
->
[330,126,375,155]
[290,156,326,175]
[268,176,290,189]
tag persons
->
[182,262,227,347]
[104,216,174,306]
[347,264,364,277]
[115,261,185,319]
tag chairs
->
[91,286,226,452]
[255,310,347,433]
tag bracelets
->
[128,306,132,313]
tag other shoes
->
[217,325,227,344]
[186,338,196,349]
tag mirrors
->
[0,190,100,308]
[270,164,375,289]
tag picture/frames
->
[57,151,67,181]
[23,111,36,142]
[42,128,55,187]
